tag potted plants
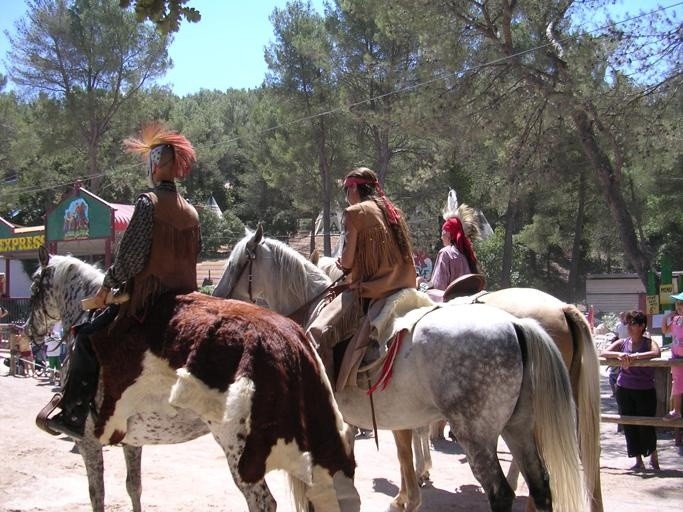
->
[0,273,7,295]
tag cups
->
[624,320,638,325]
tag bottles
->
[670,292,683,301]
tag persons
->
[612,311,631,340]
[46,122,205,441]
[0,305,67,386]
[661,292,683,421]
[601,308,661,474]
[606,337,621,398]
[303,166,419,393]
[426,186,484,288]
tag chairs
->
[663,410,681,422]
[650,459,660,470]
[625,460,645,470]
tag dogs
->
[212,222,589,512]
[22,247,360,512]
[308,249,603,512]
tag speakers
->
[47,373,96,441]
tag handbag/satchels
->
[636,351,639,359]
